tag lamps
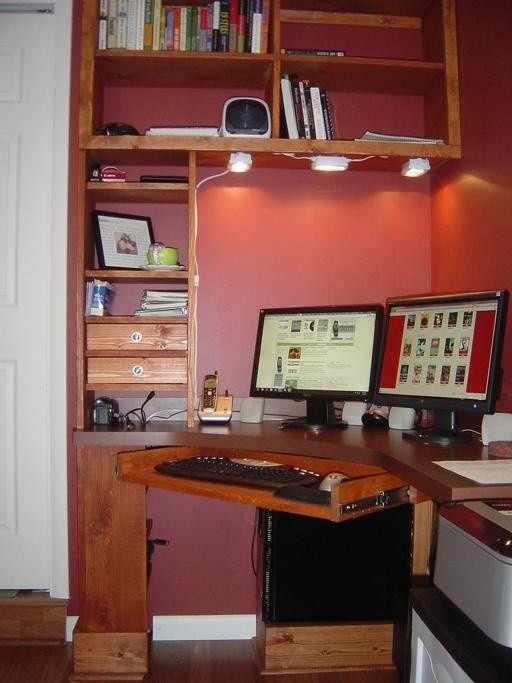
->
[226,152,431,178]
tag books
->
[279,72,336,139]
[354,138,438,144]
[146,126,220,136]
[279,47,345,57]
[134,286,189,316]
[352,130,444,143]
[94,0,270,54]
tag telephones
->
[198,371,234,424]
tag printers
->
[433,500,512,651]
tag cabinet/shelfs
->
[80,1,461,165]
[77,150,198,430]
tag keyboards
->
[154,455,323,490]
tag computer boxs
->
[254,508,415,624]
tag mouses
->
[318,471,353,492]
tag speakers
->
[221,97,272,139]
[481,412,512,445]
[240,396,265,423]
[342,401,367,425]
[388,407,416,429]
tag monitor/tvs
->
[372,288,509,444]
[249,304,385,431]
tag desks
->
[72,418,511,682]
